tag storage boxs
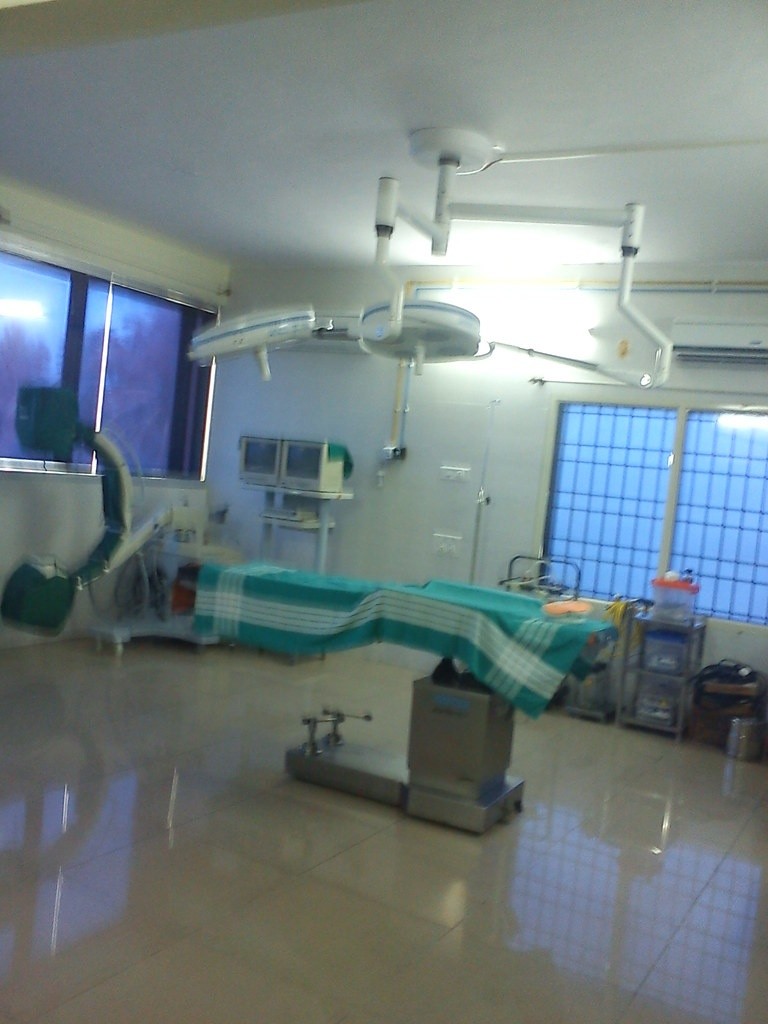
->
[644,629,700,670]
[653,578,699,619]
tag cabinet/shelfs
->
[618,614,708,744]
